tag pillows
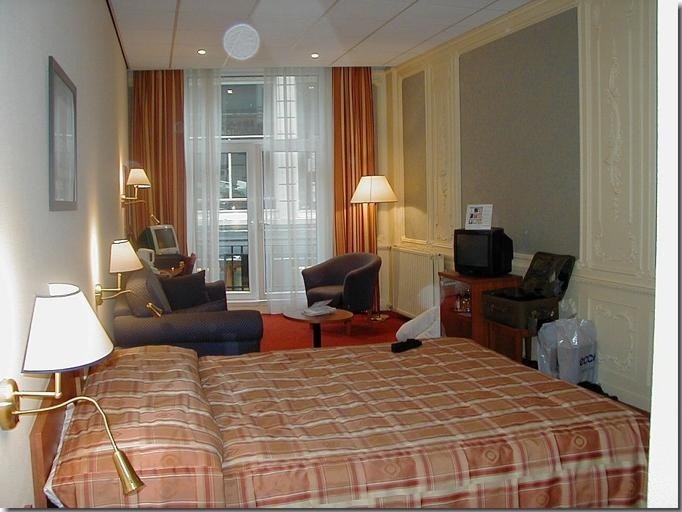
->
[158,270,211,311]
[125,270,173,317]
[45,345,223,493]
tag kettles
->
[137,248,155,267]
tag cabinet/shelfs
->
[439,271,522,352]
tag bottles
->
[454,287,472,313]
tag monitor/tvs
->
[140,224,180,255]
[453,227,514,278]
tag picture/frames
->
[48,56,79,212]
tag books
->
[302,299,336,317]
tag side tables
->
[282,309,354,348]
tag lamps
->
[0,283,144,495]
[95,239,163,318]
[350,175,399,321]
[121,168,160,224]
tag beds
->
[29,336,649,509]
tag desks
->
[150,263,185,281]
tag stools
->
[493,321,539,363]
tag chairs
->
[301,252,382,328]
[113,280,264,357]
[183,253,199,275]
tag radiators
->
[389,246,445,319]
